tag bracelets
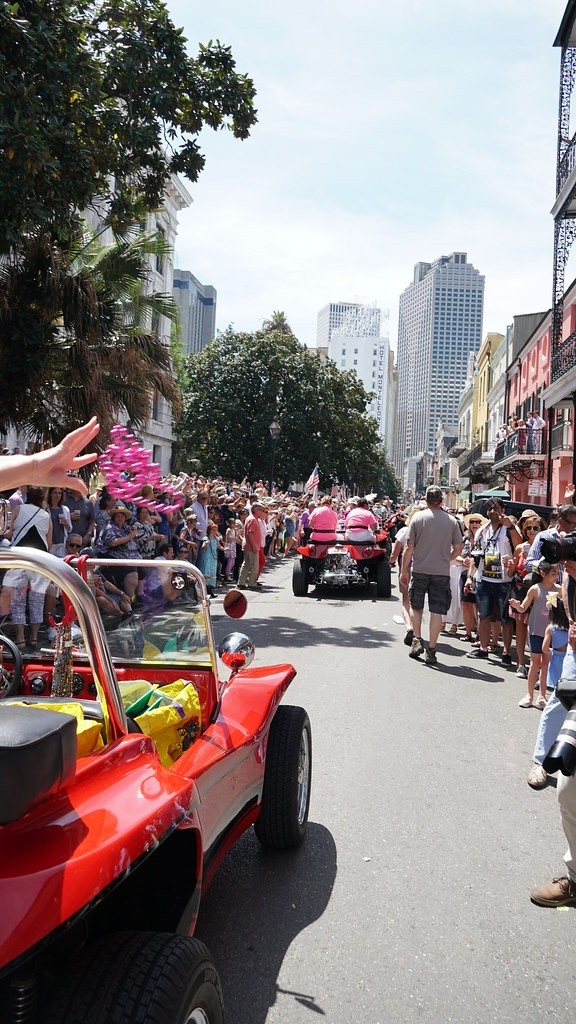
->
[120,591,124,596]
[466,575,473,580]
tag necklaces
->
[99,424,184,514]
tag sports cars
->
[0,543,314,1023]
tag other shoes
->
[436,621,511,666]
[517,667,528,677]
[135,548,295,608]
[537,696,550,709]
[9,610,141,653]
[528,764,548,785]
[405,628,415,645]
[519,696,534,708]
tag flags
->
[305,467,319,490]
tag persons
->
[0,416,100,496]
[496,410,546,456]
[0,448,576,905]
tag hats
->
[516,509,542,526]
[109,506,133,519]
[463,512,489,529]
[96,484,105,489]
[348,497,357,506]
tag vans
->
[468,499,556,525]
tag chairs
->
[1,695,144,746]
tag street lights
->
[268,417,282,497]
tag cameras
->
[539,531,576,564]
[470,549,485,568]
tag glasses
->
[468,518,482,525]
[562,518,576,524]
[525,526,540,532]
[67,541,82,548]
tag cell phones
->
[524,544,530,554]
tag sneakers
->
[425,646,437,664]
[409,639,424,657]
[530,876,576,906]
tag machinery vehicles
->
[291,520,398,598]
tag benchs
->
[305,538,375,546]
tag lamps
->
[530,462,555,477]
